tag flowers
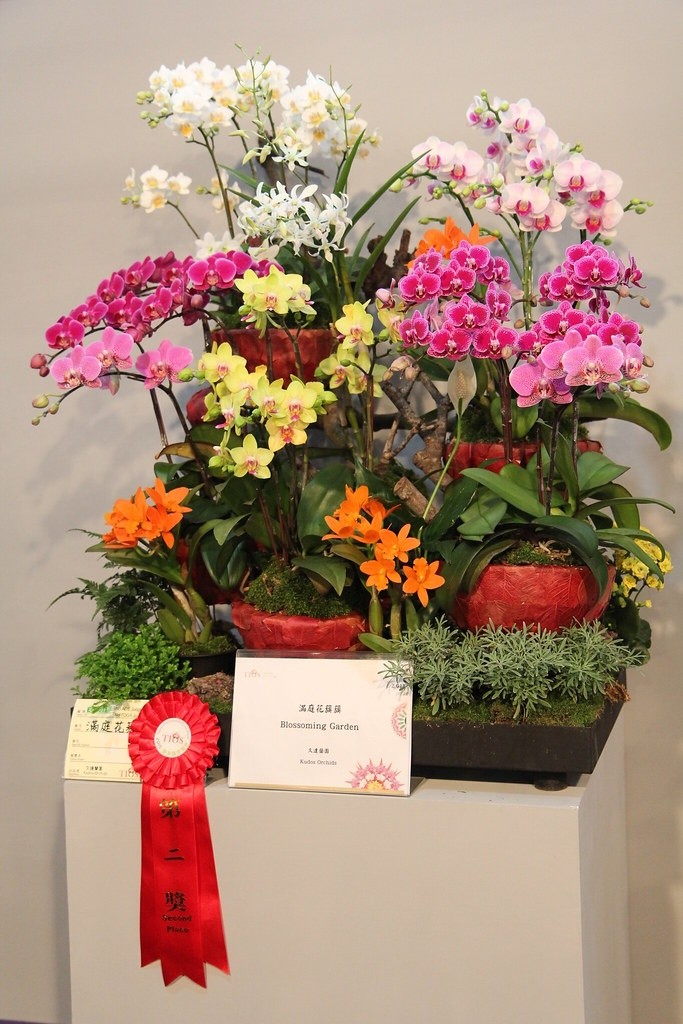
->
[30,58,679,714]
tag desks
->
[64,717,633,1024]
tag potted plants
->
[382,614,629,791]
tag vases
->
[229,599,365,652]
[445,434,604,484]
[187,387,220,426]
[437,563,599,633]
[190,560,244,604]
[208,329,335,387]
